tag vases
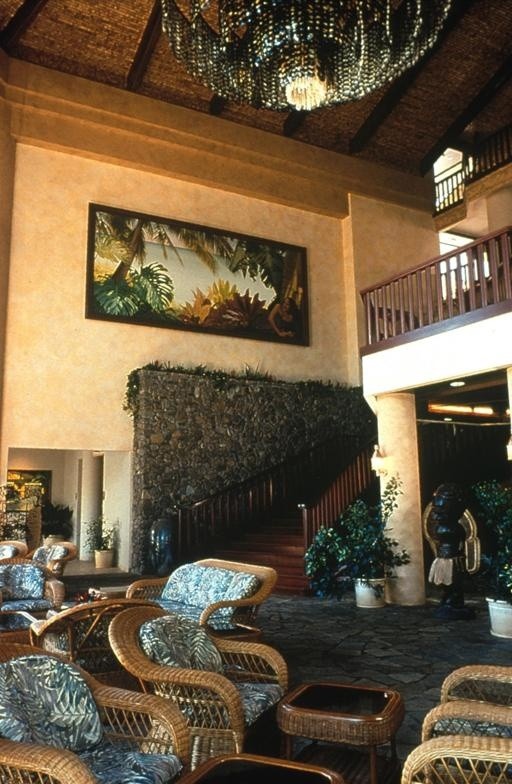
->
[149,518,174,575]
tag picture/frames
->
[85,203,309,346]
[6,469,51,512]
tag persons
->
[426,480,473,605]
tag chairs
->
[421,664,512,743]
[0,541,288,784]
[401,735,512,784]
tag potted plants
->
[83,513,115,568]
[304,472,411,608]
[472,480,512,638]
[41,503,73,546]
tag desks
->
[276,680,405,784]
[174,754,344,784]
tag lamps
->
[162,0,451,111]
